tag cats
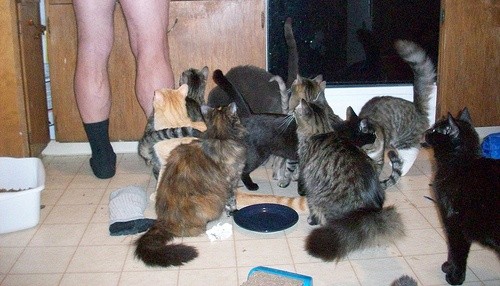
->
[128,15,440,272]
[423,106,500,286]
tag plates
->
[234,204,298,232]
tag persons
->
[72,0,176,179]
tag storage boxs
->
[0,157,46,234]
